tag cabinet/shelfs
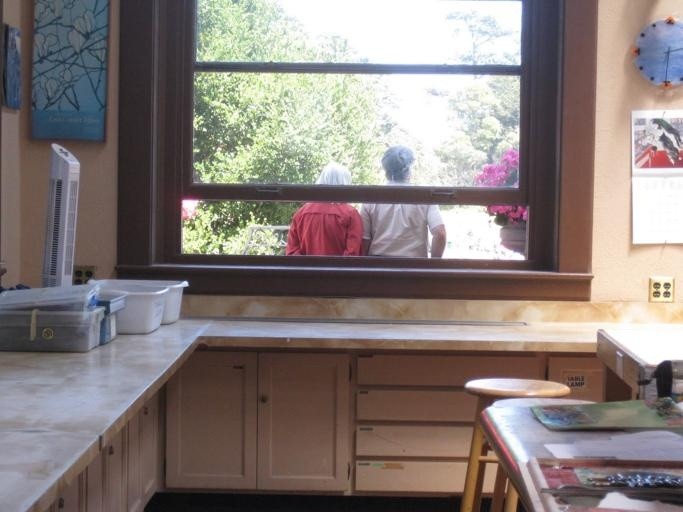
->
[548,353,606,404]
[124,385,163,511]
[49,424,124,512]
[161,351,348,491]
[348,350,545,497]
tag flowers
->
[474,148,530,225]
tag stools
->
[457,375,571,512]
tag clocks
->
[630,17,683,91]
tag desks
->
[477,394,683,512]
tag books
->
[529,394,683,432]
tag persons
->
[284,160,362,258]
[358,144,447,260]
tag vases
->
[497,220,526,251]
